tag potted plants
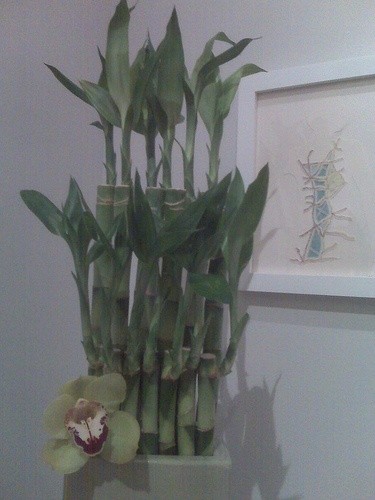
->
[22,6,265,499]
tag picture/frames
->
[231,55,374,300]
